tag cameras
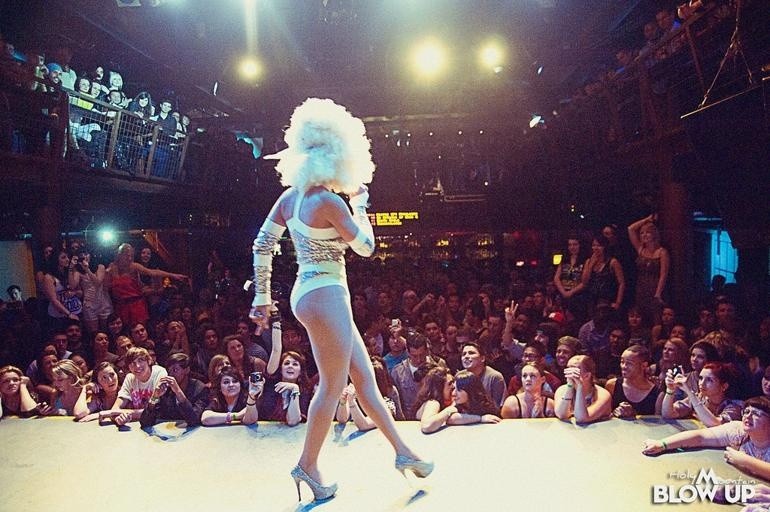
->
[250,371,263,383]
[671,366,682,378]
[76,256,85,263]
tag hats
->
[545,311,566,324]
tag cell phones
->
[392,319,398,327]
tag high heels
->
[392,453,435,480]
[289,462,341,504]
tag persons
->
[3,212,770,510]
[248,95,435,504]
[561,1,769,150]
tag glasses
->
[740,407,770,418]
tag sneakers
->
[75,149,136,175]
